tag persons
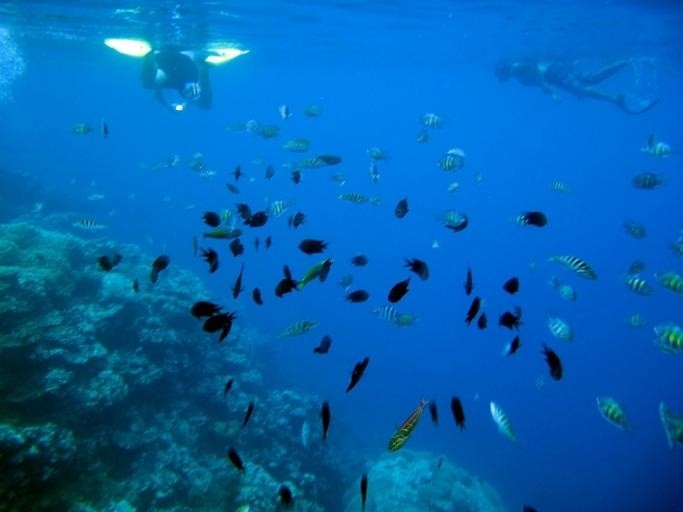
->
[497,59,660,113]
[143,8,210,108]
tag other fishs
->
[68,104,683,512]
